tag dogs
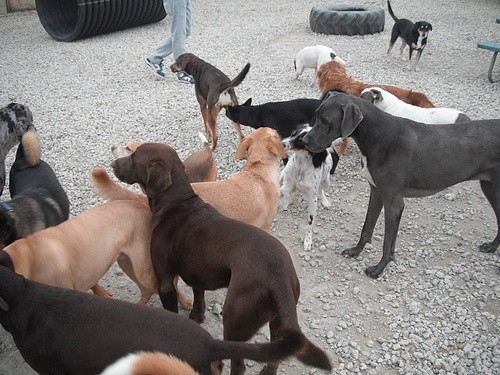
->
[0,102,35,200]
[292,44,348,95]
[278,123,345,254]
[224,96,340,176]
[316,61,436,157]
[386,0,434,73]
[358,86,472,124]
[91,125,289,314]
[110,130,218,184]
[300,90,500,280]
[0,246,306,375]
[2,197,159,305]
[0,127,113,298]
[169,51,251,153]
[110,140,333,375]
[100,348,201,375]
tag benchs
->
[478,42,500,83]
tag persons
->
[145,0,196,84]
